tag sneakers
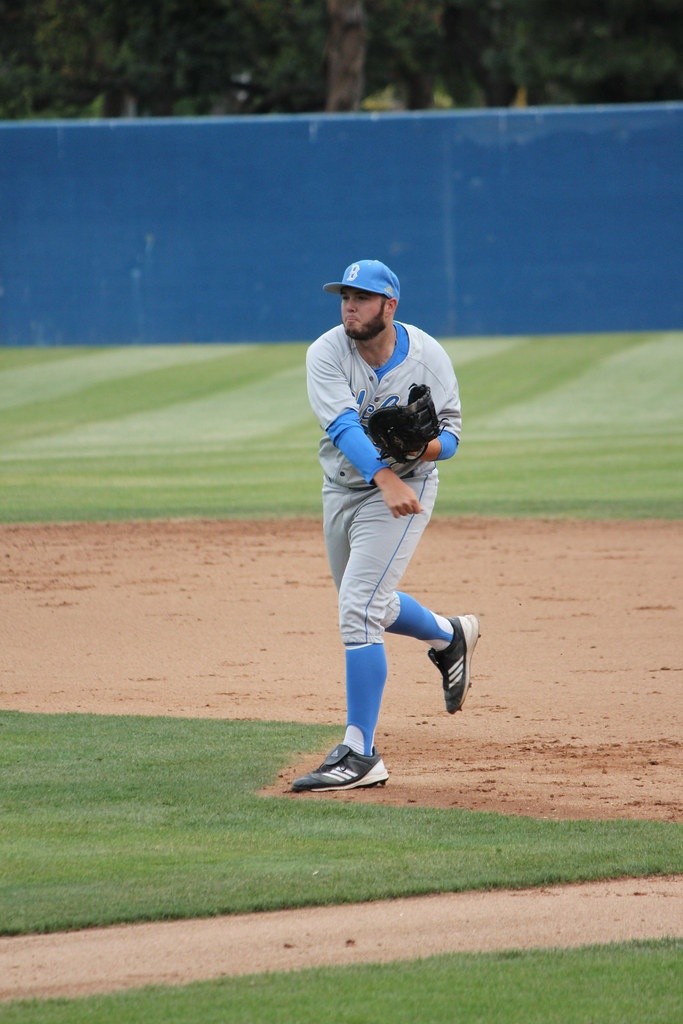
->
[429,614,479,715]
[291,744,389,792]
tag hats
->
[323,259,400,301]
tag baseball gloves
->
[367,384,441,464]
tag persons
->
[292,260,479,791]
